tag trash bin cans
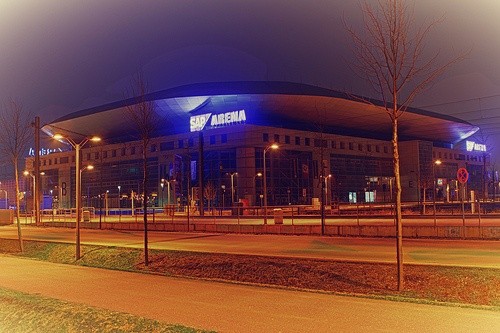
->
[331,201,338,209]
[83,210,90,222]
[184,206,190,212]
[233,202,243,215]
[274,209,283,224]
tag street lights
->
[24,170,45,217]
[52,133,101,260]
[320,174,332,206]
[264,143,279,224]
[225,171,238,207]
[432,160,441,201]
[79,165,94,215]
[161,178,176,215]
